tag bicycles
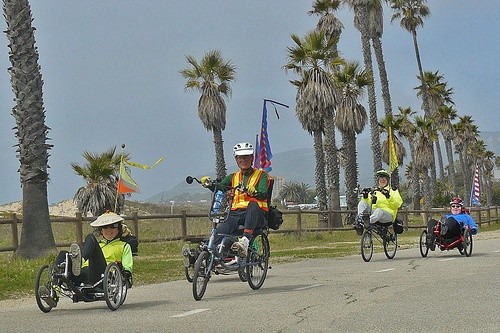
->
[352,185,401,263]
[186,173,274,302]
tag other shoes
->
[182,244,195,267]
[38,286,59,308]
[426,234,436,251]
[362,209,372,229]
[229,236,252,258]
[353,221,365,236]
[69,243,83,276]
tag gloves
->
[201,176,212,188]
[245,184,258,198]
[470,229,478,235]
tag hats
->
[90,212,125,227]
[233,142,254,156]
[450,197,464,206]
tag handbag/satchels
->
[268,205,283,230]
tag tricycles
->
[182,230,254,284]
[418,211,473,259]
[34,253,134,314]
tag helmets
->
[376,168,390,181]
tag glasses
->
[451,204,461,208]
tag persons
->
[428,196,479,247]
[353,170,403,234]
[201,142,270,264]
[53,210,133,302]
